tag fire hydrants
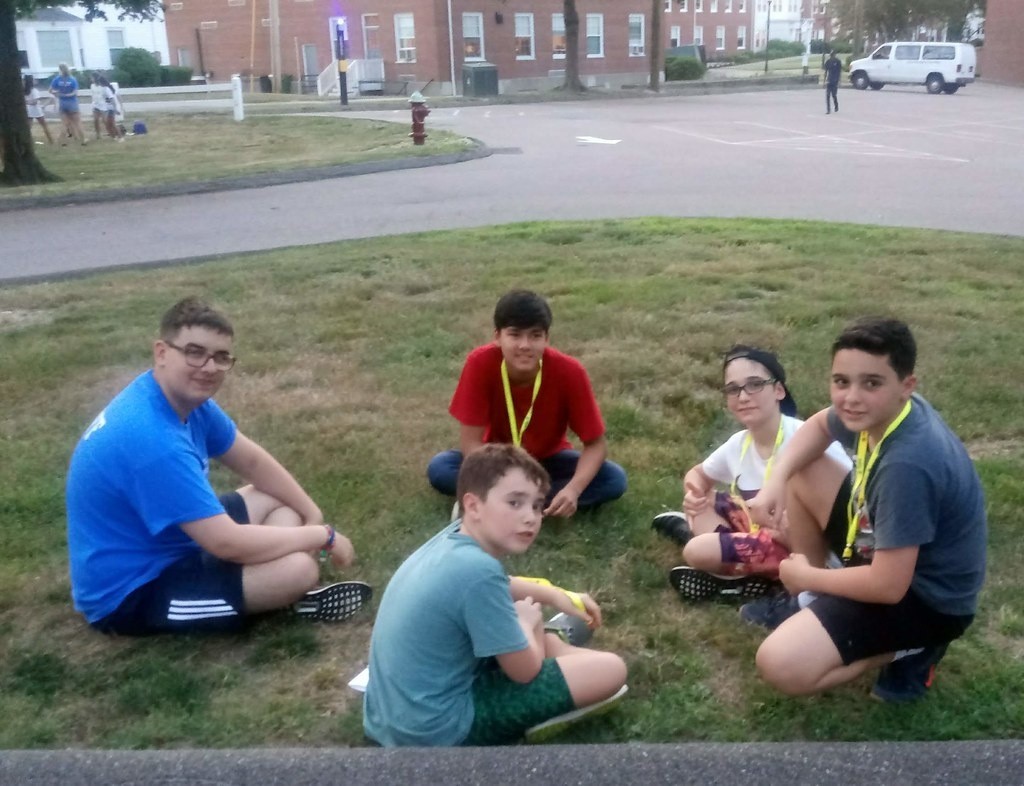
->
[406,90,433,146]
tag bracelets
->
[323,528,336,549]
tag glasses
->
[164,343,237,372]
[721,376,778,398]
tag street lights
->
[336,17,352,107]
[764,0,773,71]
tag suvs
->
[847,41,977,95]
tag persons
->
[25,88,53,143]
[744,323,985,703]
[364,443,630,748]
[48,63,85,145]
[65,294,376,633]
[652,345,855,604]
[427,289,628,520]
[91,72,128,142]
[823,50,842,114]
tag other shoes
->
[652,510,694,545]
[670,567,766,604]
[278,580,375,627]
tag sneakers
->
[739,596,801,631]
[547,610,592,647]
[526,684,629,743]
[869,646,946,704]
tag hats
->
[722,346,798,418]
